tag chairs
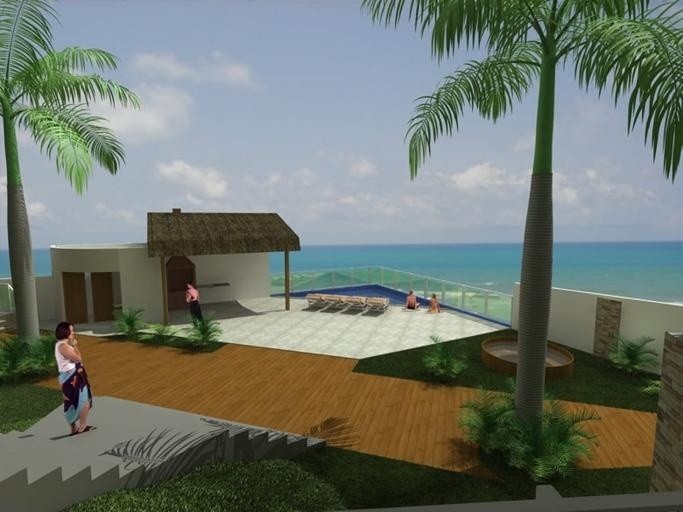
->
[305,293,389,312]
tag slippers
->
[68,425,97,436]
